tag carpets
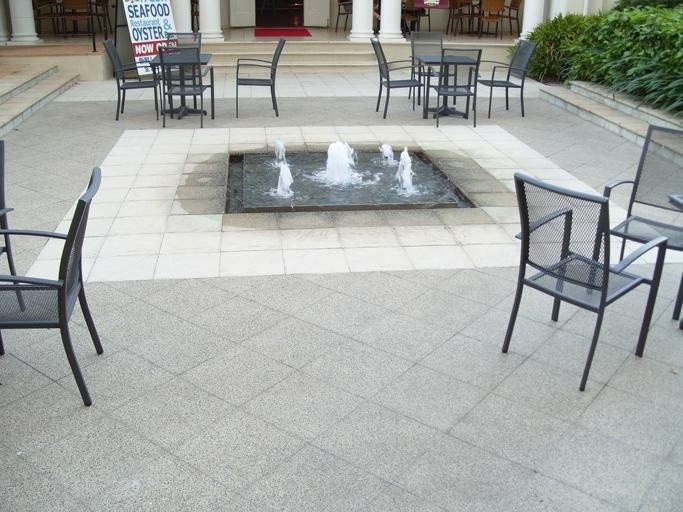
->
[255,29,311,37]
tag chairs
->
[500,172,670,393]
[334,0,538,127]
[586,123,683,320]
[32,0,285,128]
[0,166,103,407]
[0,139,26,313]
